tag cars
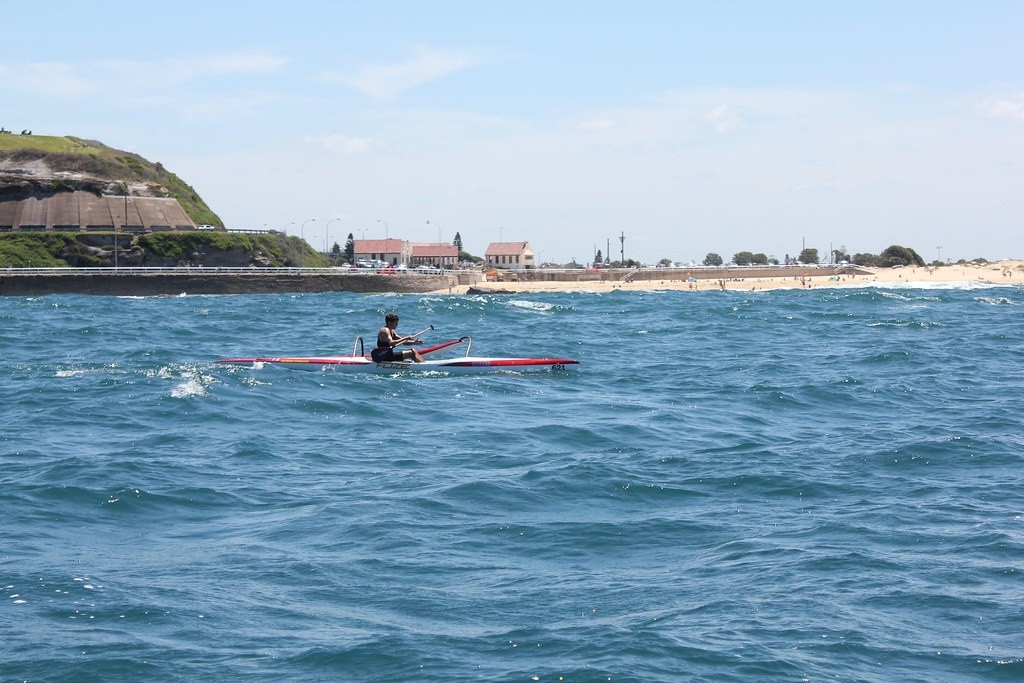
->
[356,259,389,268]
[198,225,215,230]
[393,264,440,274]
[342,263,351,267]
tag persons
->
[681,272,877,292]
[377,313,426,363]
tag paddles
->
[369,324,435,363]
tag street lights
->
[326,218,340,259]
[314,236,334,252]
[427,222,441,269]
[285,222,295,234]
[378,220,388,261]
[357,229,368,240]
[937,247,942,262]
[301,219,316,267]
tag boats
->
[214,355,581,372]
